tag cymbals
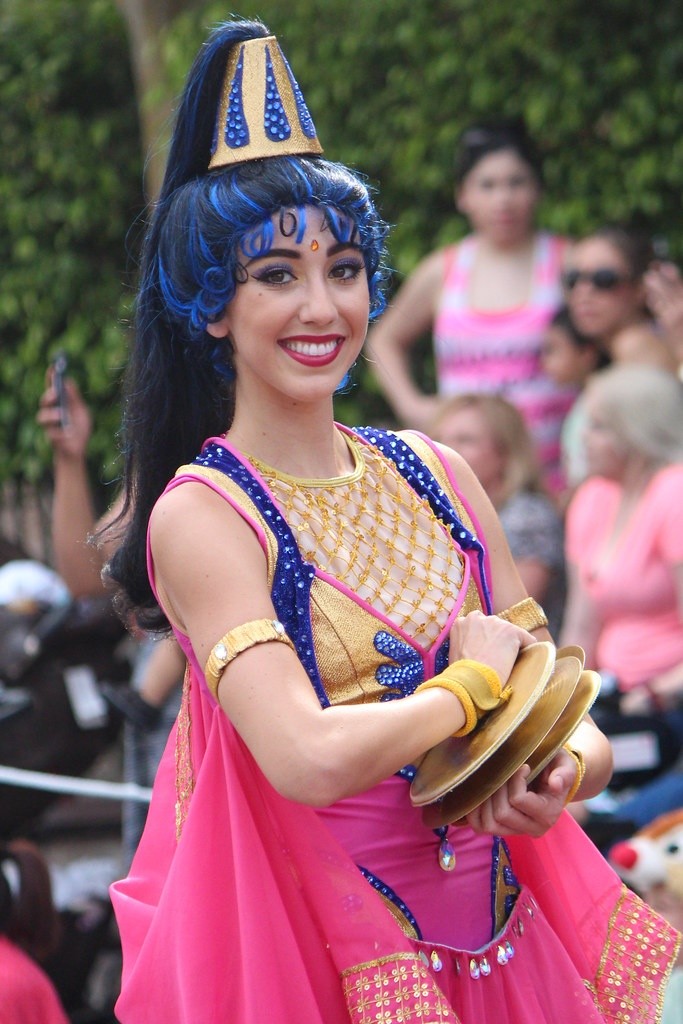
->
[409,639,605,832]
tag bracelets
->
[644,683,665,718]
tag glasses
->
[562,267,636,289]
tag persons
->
[37,358,185,850]
[110,22,680,1023]
[362,110,683,716]
[1,837,70,1023]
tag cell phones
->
[54,359,69,428]
